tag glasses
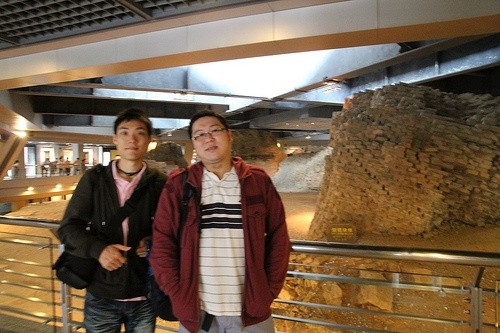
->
[190,127,227,140]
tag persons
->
[150,110,292,333]
[43,155,91,175]
[57,107,168,333]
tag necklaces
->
[116,161,143,176]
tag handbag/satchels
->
[149,276,179,322]
[50,251,97,289]
